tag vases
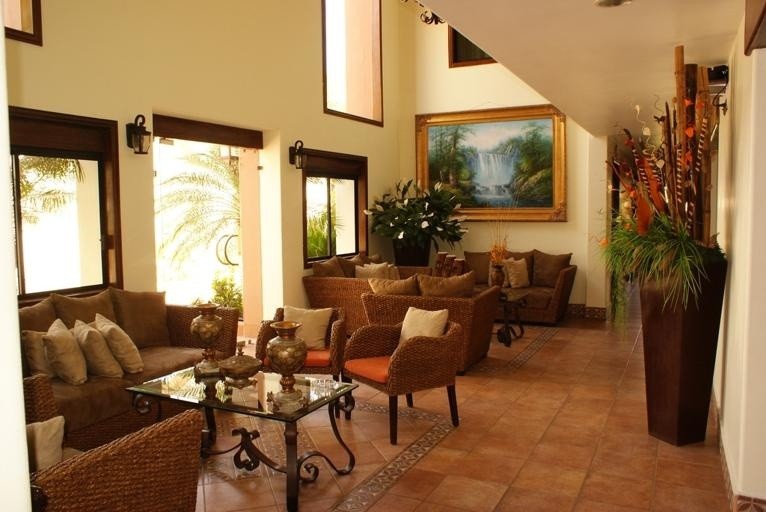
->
[391,234,431,266]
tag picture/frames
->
[414,103,568,222]
[447,23,498,67]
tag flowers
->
[364,176,468,255]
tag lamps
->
[126,114,151,155]
[288,142,307,169]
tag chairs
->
[341,318,461,446]
[256,306,343,418]
[304,265,431,337]
[25,410,204,512]
[361,287,501,375]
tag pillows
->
[487,258,526,289]
[27,414,73,473]
[283,307,333,350]
[357,263,477,297]
[23,314,146,384]
[398,306,449,341]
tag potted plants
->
[595,44,728,449]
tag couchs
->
[429,249,576,328]
[19,288,239,434]
